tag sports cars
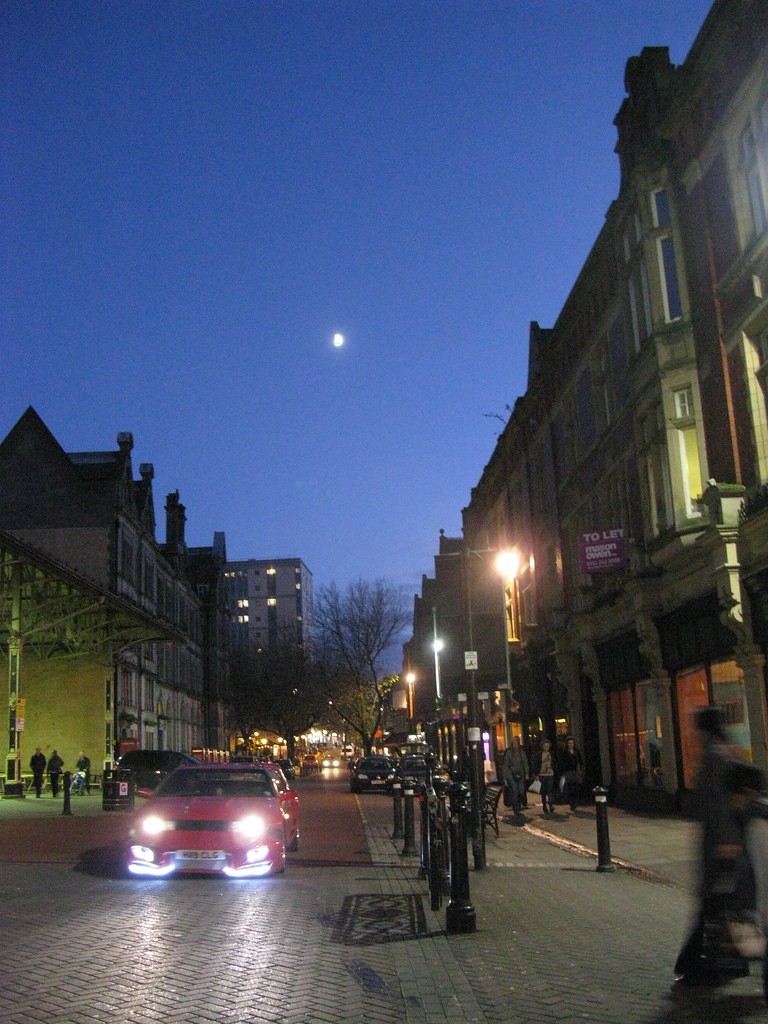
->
[123,764,296,879]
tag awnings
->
[380,734,406,748]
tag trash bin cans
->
[102,767,135,813]
[448,784,468,817]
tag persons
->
[46,750,64,797]
[75,751,91,796]
[29,748,48,798]
[669,705,768,1003]
[561,736,585,812]
[347,757,355,771]
[533,740,558,813]
[460,746,471,768]
[501,736,534,815]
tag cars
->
[302,755,319,767]
[309,746,318,754]
[113,750,208,797]
[350,756,397,793]
[322,755,335,767]
[340,747,354,759]
[396,754,450,795]
[208,763,300,852]
[274,759,295,779]
[228,756,271,763]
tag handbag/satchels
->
[558,775,566,794]
[674,896,768,978]
[528,778,542,794]
[512,771,523,782]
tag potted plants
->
[737,482,768,545]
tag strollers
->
[70,770,86,796]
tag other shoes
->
[542,807,548,812]
[570,805,577,810]
[549,807,555,812]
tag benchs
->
[449,780,503,836]
